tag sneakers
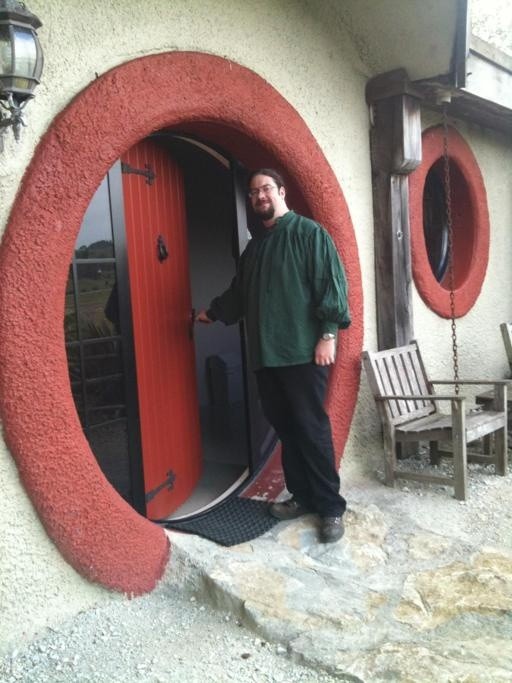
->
[269,497,317,520]
[321,515,344,542]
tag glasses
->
[248,186,277,197]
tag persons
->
[193,170,352,544]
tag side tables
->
[475,379,512,466]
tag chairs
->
[360,340,512,500]
[499,322,512,374]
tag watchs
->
[319,333,335,341]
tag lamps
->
[0,0,44,154]
